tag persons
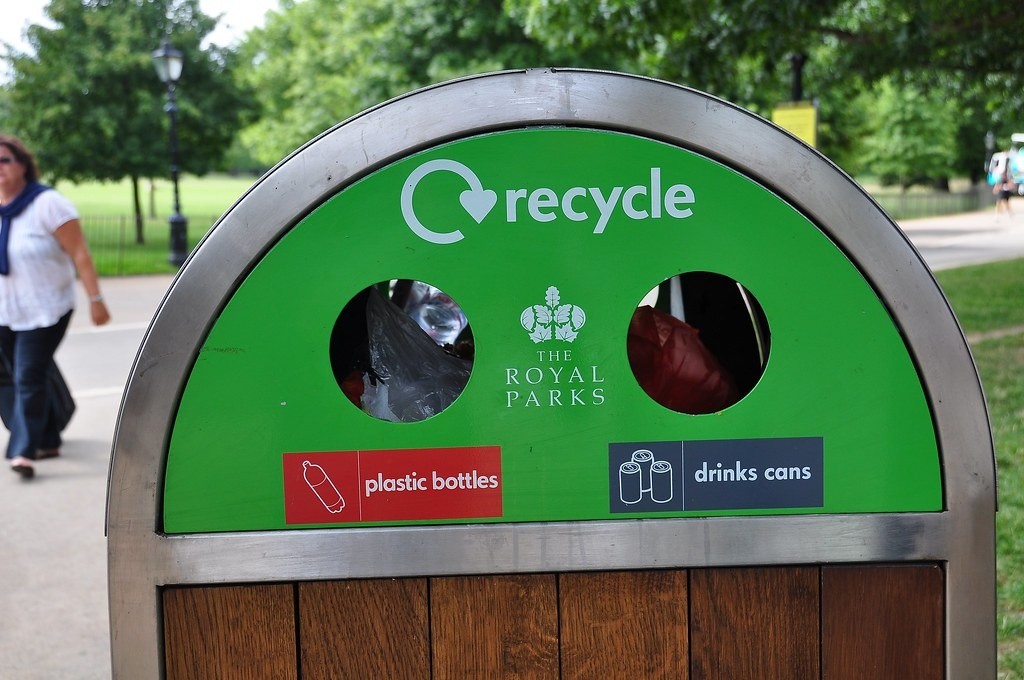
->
[0,135,110,478]
[991,156,1016,220]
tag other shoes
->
[8,454,34,476]
[31,445,60,459]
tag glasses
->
[0,157,18,167]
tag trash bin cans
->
[102,65,1002,680]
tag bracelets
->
[89,295,103,302]
